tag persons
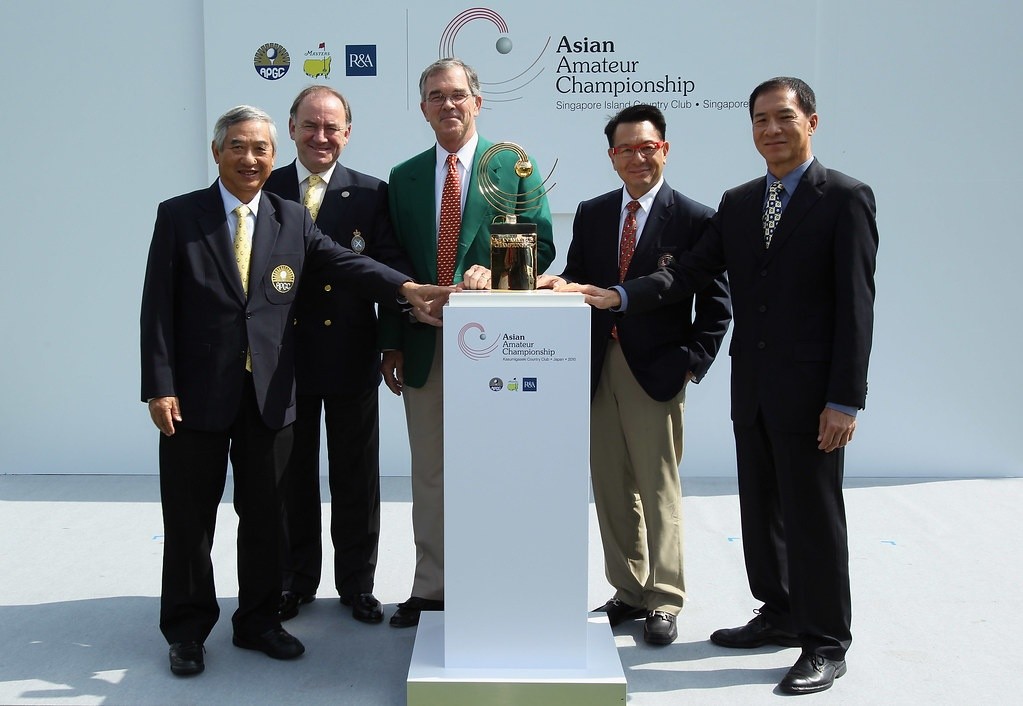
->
[537,105,731,646]
[378,59,555,629]
[141,105,462,676]
[551,77,878,695]
[260,87,389,622]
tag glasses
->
[608,141,664,160]
[424,93,478,106]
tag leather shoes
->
[780,648,846,693]
[389,596,444,628]
[168,638,207,676]
[233,625,305,660]
[644,609,678,644]
[591,598,648,627]
[340,590,385,624]
[711,609,801,648]
[278,590,317,623]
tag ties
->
[611,201,641,342]
[762,180,784,249]
[437,153,459,286]
[303,175,323,223]
[232,205,253,373]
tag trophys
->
[476,142,559,291]
